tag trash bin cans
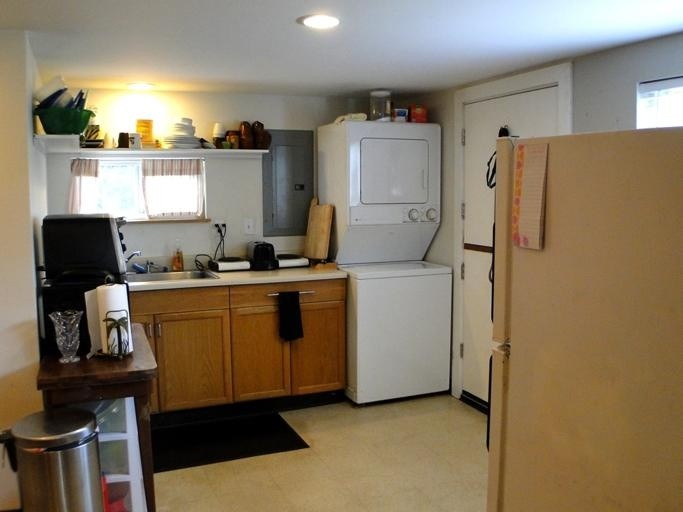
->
[0,409,103,512]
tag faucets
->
[124,250,142,264]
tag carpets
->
[151,392,344,473]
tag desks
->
[36,323,158,512]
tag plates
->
[164,123,200,148]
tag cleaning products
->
[171,238,185,271]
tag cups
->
[48,310,83,363]
[116,115,152,151]
[213,123,239,148]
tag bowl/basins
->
[33,73,95,134]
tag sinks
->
[126,269,221,282]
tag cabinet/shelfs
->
[130,309,233,416]
[229,278,346,403]
